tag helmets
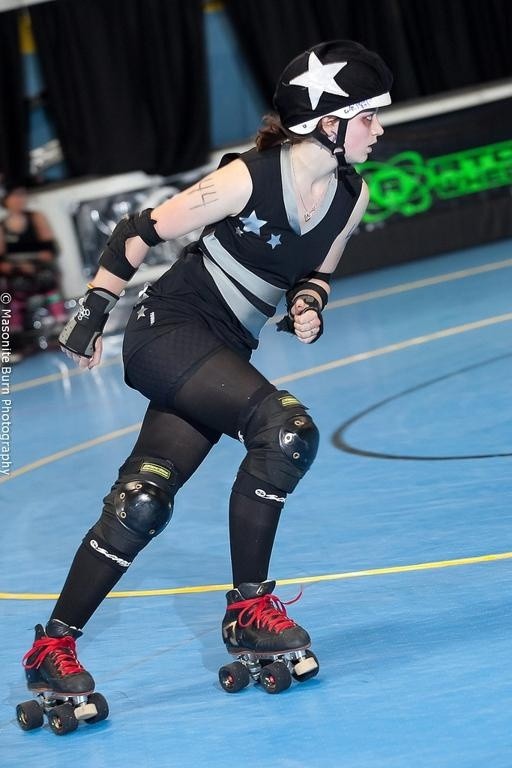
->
[273,40,395,139]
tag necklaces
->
[290,145,333,222]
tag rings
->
[310,330,313,336]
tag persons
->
[21,40,393,697]
[0,180,60,364]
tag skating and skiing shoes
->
[218,579,320,695]
[16,616,109,736]
